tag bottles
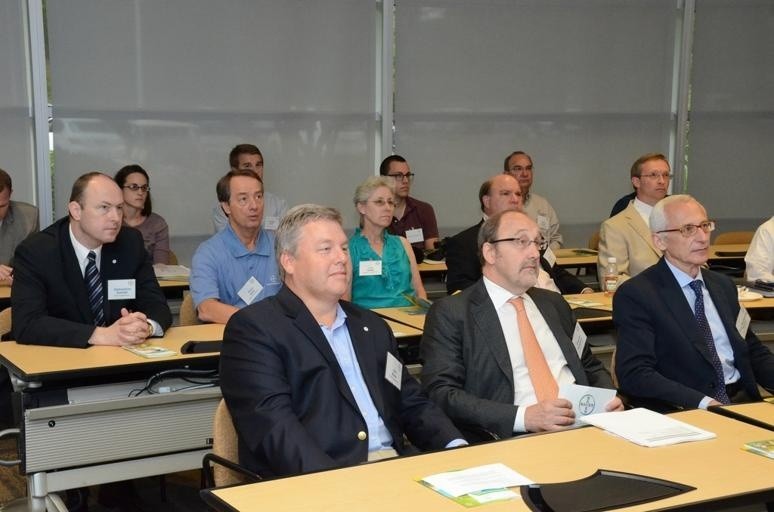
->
[603,257,619,297]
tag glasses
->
[487,238,540,248]
[385,174,415,182]
[638,173,670,180]
[358,200,394,207]
[656,222,715,237]
[122,185,150,192]
[508,167,534,172]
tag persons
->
[218,203,468,473]
[613,194,774,410]
[11,174,174,347]
[446,174,592,295]
[597,154,683,293]
[340,178,427,309]
[113,164,171,267]
[189,169,285,324]
[742,218,773,285]
[504,151,562,253]
[421,208,630,434]
[212,143,289,233]
[0,170,41,287]
[360,155,439,250]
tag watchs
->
[148,322,153,337]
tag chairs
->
[203,395,267,488]
[709,231,755,274]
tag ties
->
[689,280,732,407]
[507,297,559,403]
[84,252,107,329]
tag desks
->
[0,276,191,298]
[415,247,598,272]
[705,400,773,429]
[370,291,774,356]
[0,312,426,511]
[197,408,773,511]
[706,244,752,259]
